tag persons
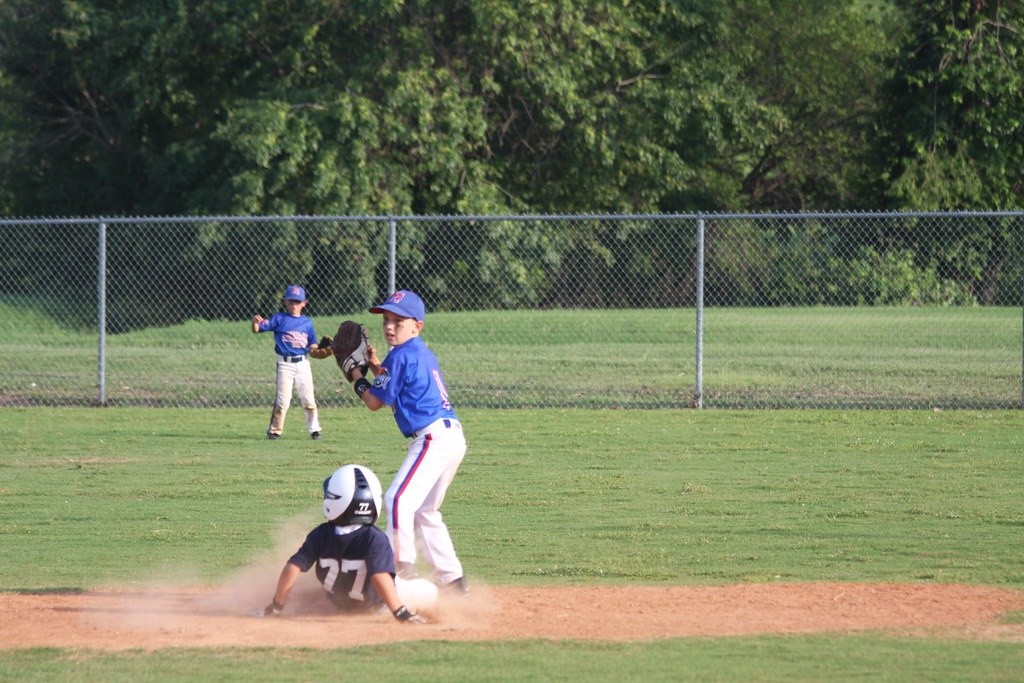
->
[263,464,439,625]
[252,285,333,440]
[332,290,471,598]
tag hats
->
[285,286,306,302]
[368,291,425,322]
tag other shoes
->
[392,560,418,580]
[311,431,320,440]
[436,577,469,598]
[268,433,280,439]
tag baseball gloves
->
[331,321,371,382]
[309,336,334,360]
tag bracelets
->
[393,605,411,623]
[353,377,371,397]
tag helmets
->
[321,465,382,526]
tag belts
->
[411,420,452,439]
[283,356,302,363]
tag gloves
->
[393,606,428,625]
[246,599,283,617]
